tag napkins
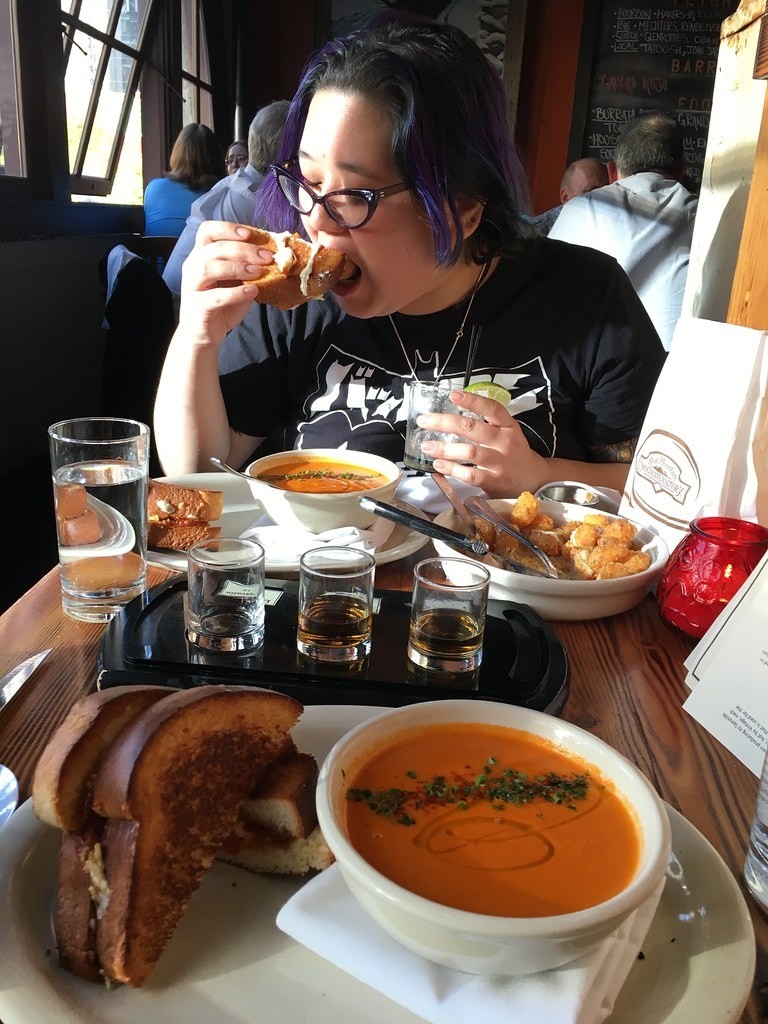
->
[276,858,668,1023]
[239,507,396,561]
[394,460,489,514]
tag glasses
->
[271,158,412,229]
[224,156,246,166]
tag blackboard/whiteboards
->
[568,0,739,193]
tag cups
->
[407,556,490,671]
[187,540,266,653]
[403,379,485,476]
[296,545,376,660]
[50,422,149,624]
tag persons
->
[224,140,249,176]
[143,122,224,278]
[545,110,699,353]
[154,11,670,500]
[530,158,609,238]
[161,100,293,326]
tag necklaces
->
[388,259,486,386]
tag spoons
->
[463,501,584,581]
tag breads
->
[29,685,333,991]
[54,482,102,546]
[146,479,224,551]
[231,224,357,311]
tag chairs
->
[100,235,177,393]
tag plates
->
[142,474,429,573]
[0,705,755,1024]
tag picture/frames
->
[313,0,528,148]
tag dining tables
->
[0,510,767,1023]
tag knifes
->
[360,498,557,581]
[0,648,53,712]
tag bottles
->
[657,517,768,638]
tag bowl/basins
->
[315,700,670,975]
[243,449,400,533]
[542,486,600,507]
[433,499,667,621]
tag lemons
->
[459,382,511,410]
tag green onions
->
[341,757,594,826]
[256,468,352,485]
